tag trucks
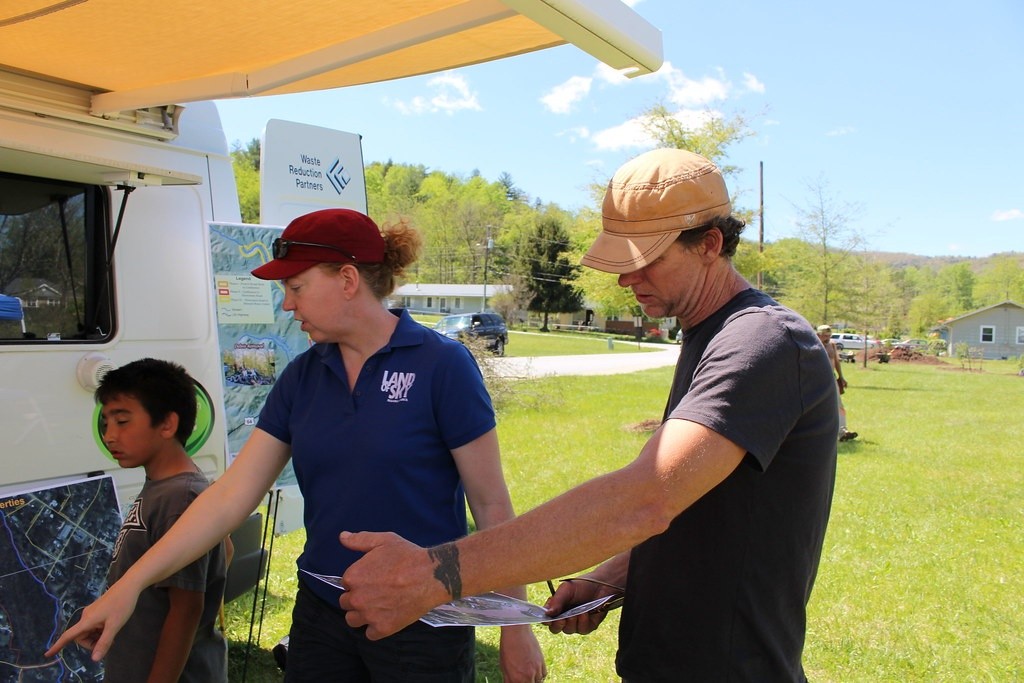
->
[0,0,666,608]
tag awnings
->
[0,0,664,116]
[0,137,203,267]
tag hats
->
[250,208,386,280]
[580,149,732,275]
[816,324,831,336]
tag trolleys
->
[876,350,891,363]
[838,352,856,363]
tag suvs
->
[431,312,509,357]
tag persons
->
[45,209,547,683]
[338,148,859,683]
[94,359,229,683]
[815,325,858,441]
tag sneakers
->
[840,431,858,442]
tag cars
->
[829,333,876,351]
[895,339,929,351]
[879,339,901,349]
[676,328,684,344]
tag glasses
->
[271,237,356,260]
